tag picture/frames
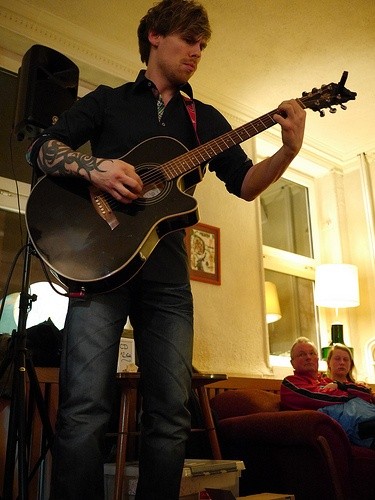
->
[185,223,222,285]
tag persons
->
[323,343,356,382]
[281,336,374,445]
[27,0,309,499]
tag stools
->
[111,371,228,500]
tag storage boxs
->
[104,458,246,500]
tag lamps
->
[314,263,360,361]
[265,281,282,323]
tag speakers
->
[15,43,79,139]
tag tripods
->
[0,128,62,498]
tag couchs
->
[216,411,375,500]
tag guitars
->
[24,71,358,299]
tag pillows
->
[209,388,281,419]
